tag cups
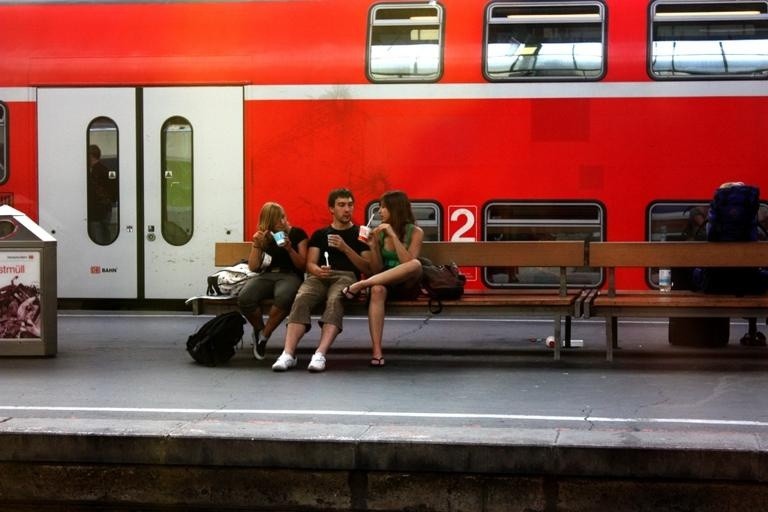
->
[358,225,371,243]
[273,231,287,248]
[327,235,336,247]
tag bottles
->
[659,268,672,292]
[545,335,555,349]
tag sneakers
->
[252,330,268,361]
[271,350,298,371]
[307,352,327,371]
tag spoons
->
[323,251,330,267]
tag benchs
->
[189,242,767,361]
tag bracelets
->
[253,245,261,249]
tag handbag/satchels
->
[418,256,465,302]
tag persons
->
[237,202,309,360]
[340,190,425,366]
[491,215,520,283]
[271,189,373,371]
[88,143,118,241]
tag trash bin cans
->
[666,233,730,350]
[0,204,57,356]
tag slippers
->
[369,357,385,367]
[341,286,356,301]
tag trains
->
[2,5,763,309]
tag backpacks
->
[206,263,252,297]
[186,312,245,368]
